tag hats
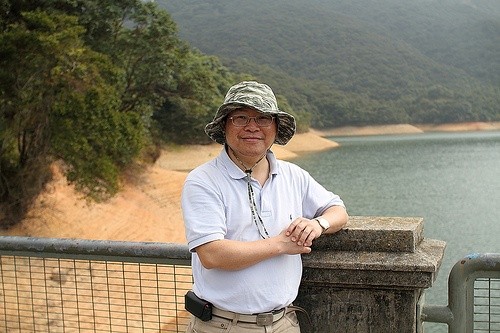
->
[204,80,297,147]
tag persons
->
[182,81,349,333]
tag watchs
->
[314,216,330,234]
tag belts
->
[211,306,316,330]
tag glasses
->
[225,110,278,127]
